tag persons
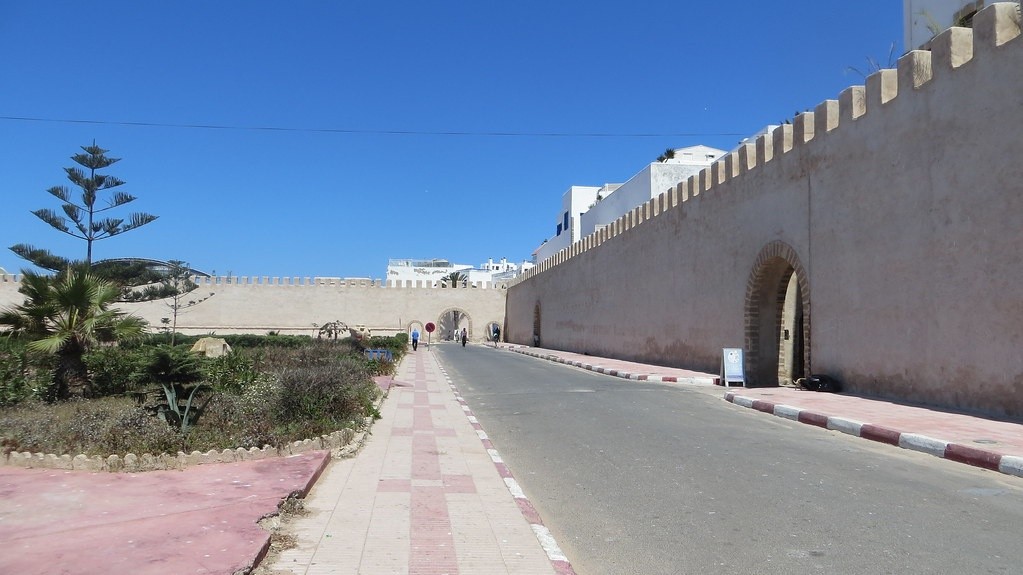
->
[412,328,419,351]
[455,333,459,343]
[462,328,466,346]
[495,327,500,342]
[448,330,451,337]
